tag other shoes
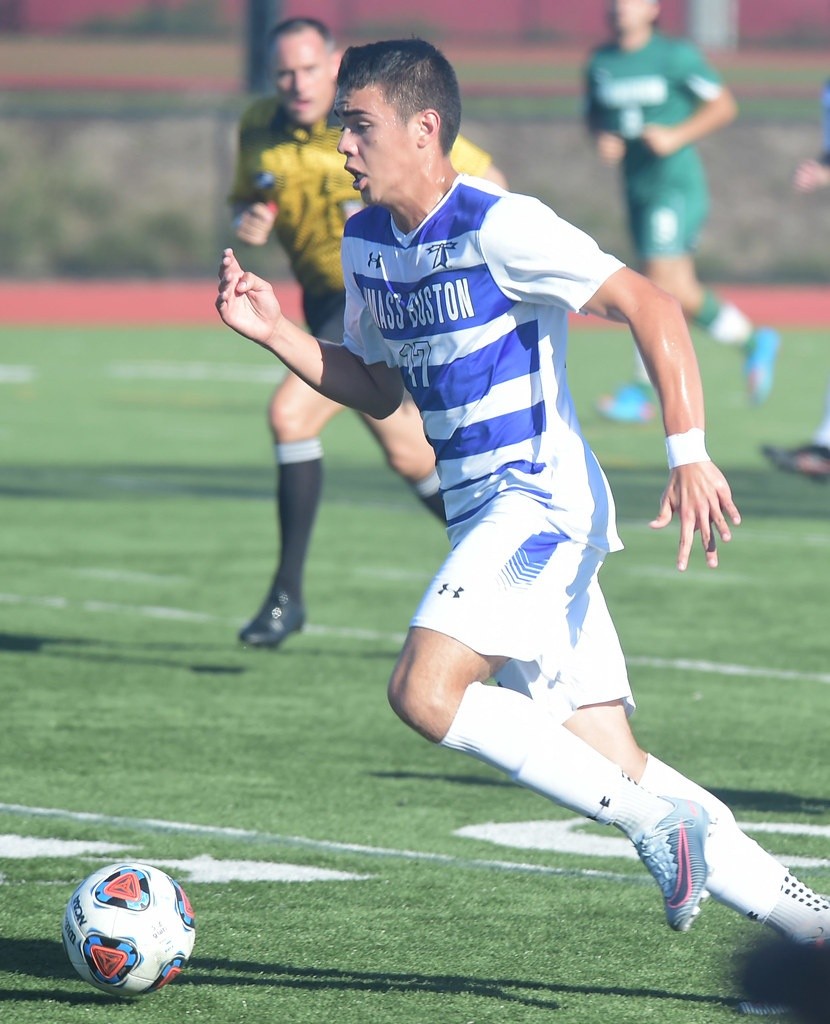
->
[241,596,305,645]
[761,443,830,480]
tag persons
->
[229,17,505,651]
[216,39,830,1015]
[764,72,830,478]
[583,0,775,423]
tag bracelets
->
[664,428,710,469]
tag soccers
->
[62,861,196,998]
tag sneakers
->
[630,795,715,934]
[743,328,781,402]
[737,897,830,1016]
[597,385,656,422]
